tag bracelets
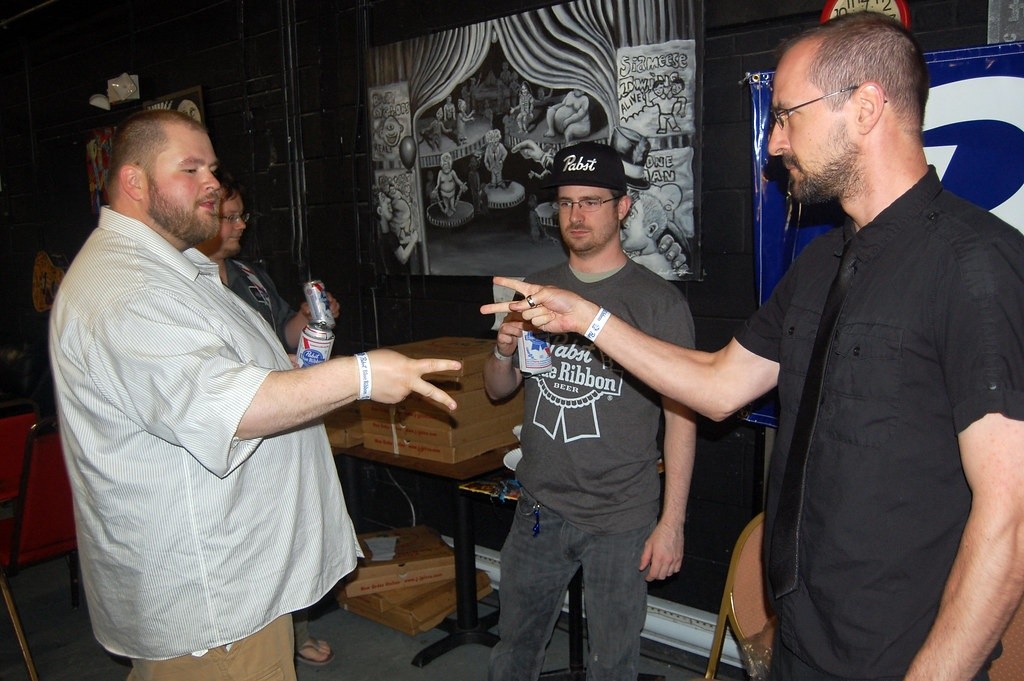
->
[584,308,612,341]
[494,346,513,360]
[355,351,372,399]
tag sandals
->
[294,634,336,667]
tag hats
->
[544,142,626,197]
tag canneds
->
[296,321,335,367]
[518,329,553,375]
[303,280,336,328]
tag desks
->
[340,445,521,667]
[456,464,668,681]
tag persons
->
[31,218,80,312]
[48,109,462,681]
[478,8,1024,681]
[482,141,697,681]
[207,170,340,666]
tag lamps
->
[90,73,140,110]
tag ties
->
[767,238,858,602]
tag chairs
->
[689,511,1024,681]
[0,399,83,681]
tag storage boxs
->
[324,336,523,464]
[338,526,496,637]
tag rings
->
[526,294,536,309]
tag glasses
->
[769,86,889,131]
[219,211,251,225]
[550,195,630,213]
